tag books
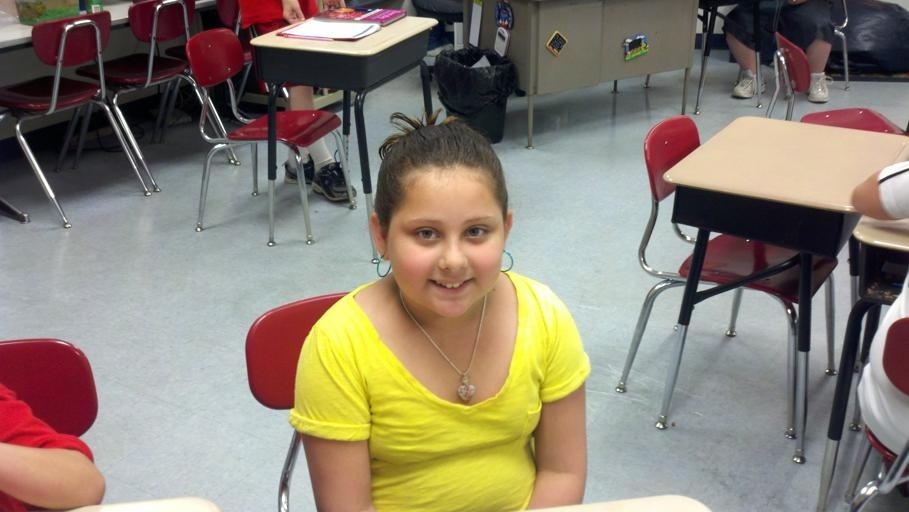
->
[314,7,408,26]
[277,18,379,41]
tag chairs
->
[1,10,153,229]
[243,291,379,512]
[0,335,102,442]
[798,105,901,133]
[185,26,358,248]
[613,114,841,467]
[53,2,235,194]
[765,30,812,120]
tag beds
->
[844,305,909,511]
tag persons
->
[285,114,593,512]
[722,2,838,105]
[0,381,106,511]
[237,1,357,203]
[854,161,909,456]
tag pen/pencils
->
[281,34,335,44]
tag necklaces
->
[390,285,493,401]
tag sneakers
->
[284,153,315,185]
[311,162,357,202]
[731,68,765,99]
[806,71,834,103]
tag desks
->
[655,113,908,462]
[250,6,439,265]
[813,131,909,511]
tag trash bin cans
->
[436,47,513,143]
